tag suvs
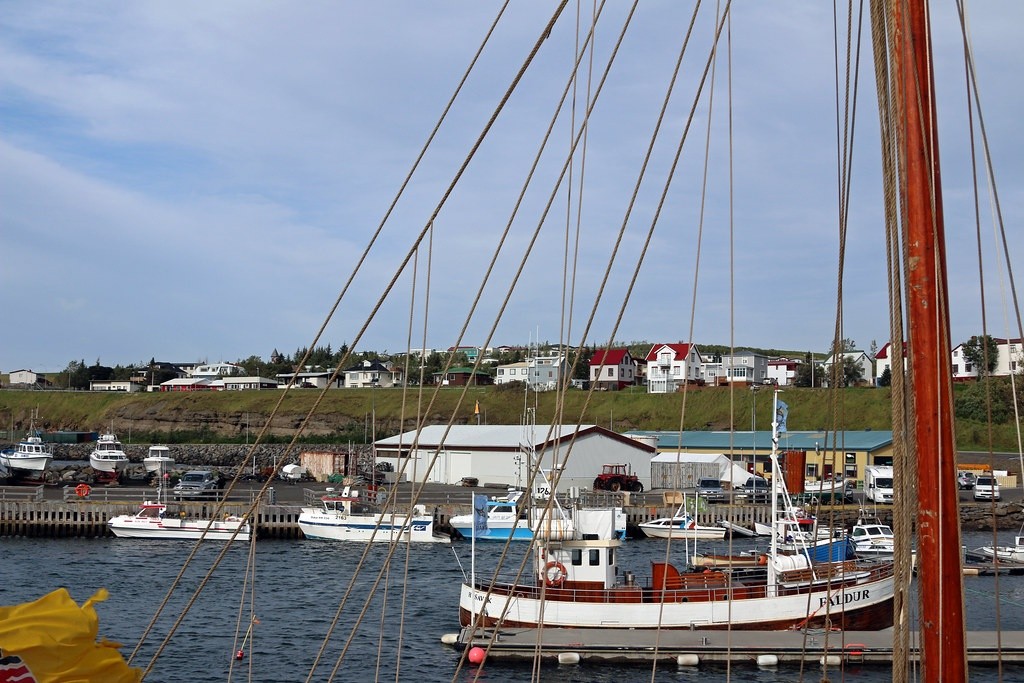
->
[695,476,726,505]
[957,471,976,490]
[741,476,771,502]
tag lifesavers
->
[542,561,567,585]
[76,484,88,497]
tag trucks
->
[862,464,893,505]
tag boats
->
[638,510,726,541]
[850,497,918,568]
[142,444,176,474]
[776,529,858,564]
[106,459,251,541]
[0,409,54,480]
[89,419,130,479]
[754,490,821,542]
[295,486,451,545]
[804,478,849,502]
[440,381,922,665]
[448,488,575,544]
[982,519,1024,567]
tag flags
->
[776,400,788,432]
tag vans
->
[973,475,1004,502]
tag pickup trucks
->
[173,470,219,501]
